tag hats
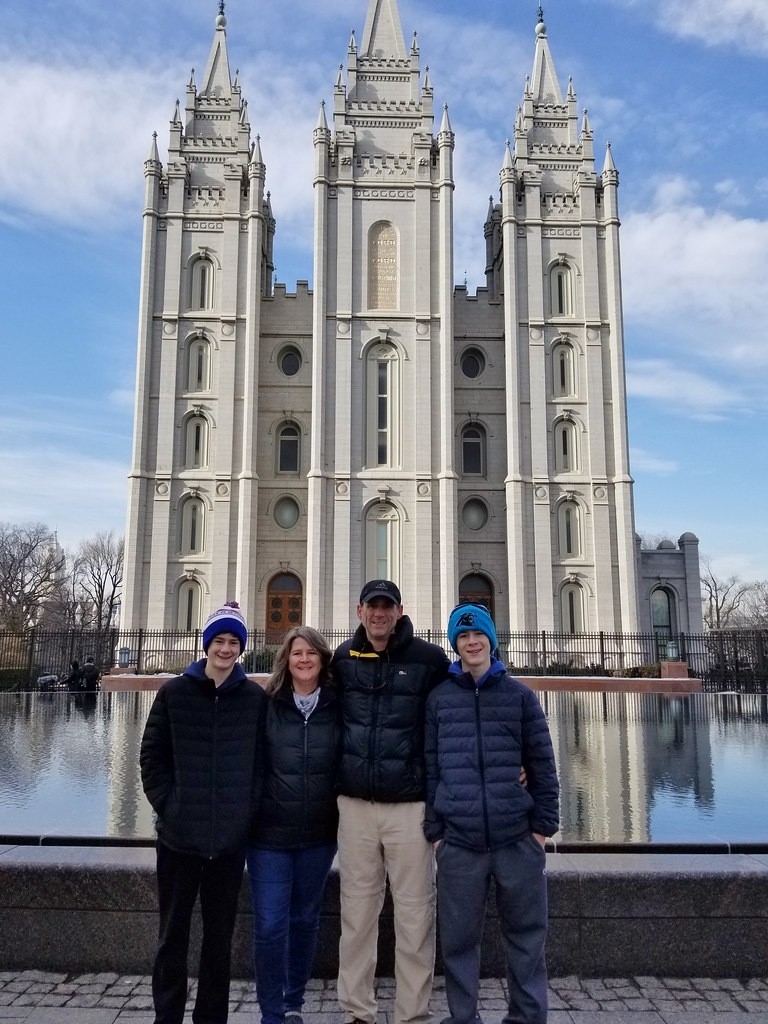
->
[359,580,401,607]
[447,602,497,655]
[203,601,247,658]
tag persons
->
[60,657,99,691]
[246,626,340,1024]
[329,579,527,1024]
[424,603,561,1024]
[140,602,264,1024]
